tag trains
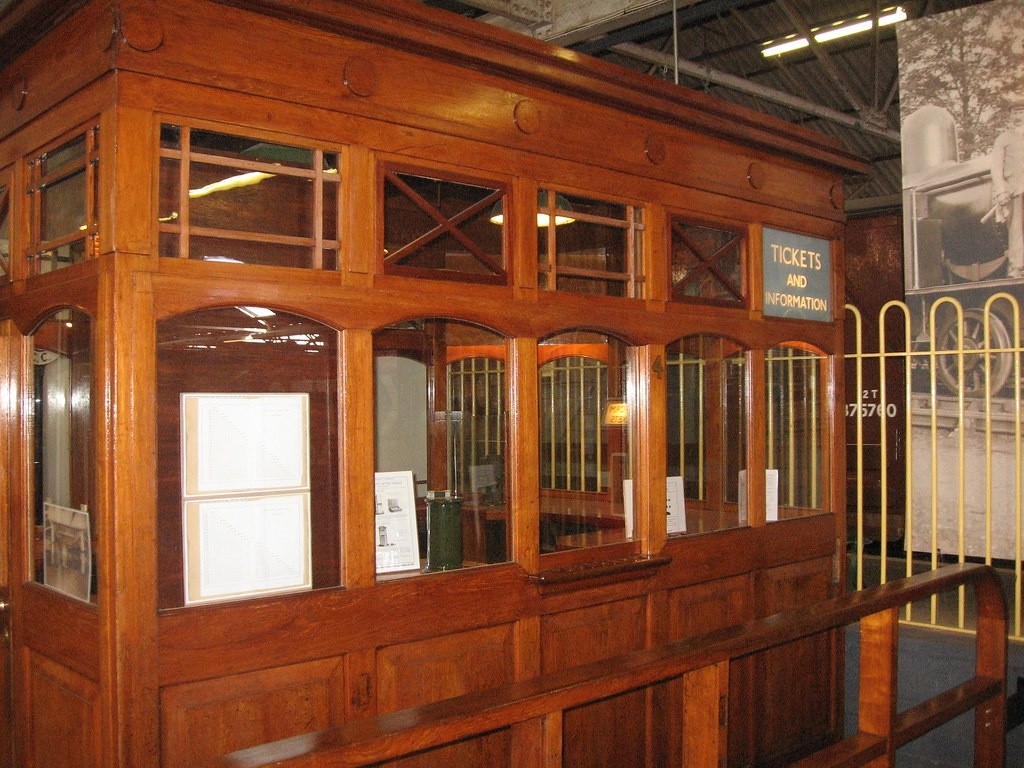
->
[900,102,1024,400]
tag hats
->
[1007,100,1024,111]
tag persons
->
[991,100,1024,279]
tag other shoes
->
[1007,269,1024,279]
